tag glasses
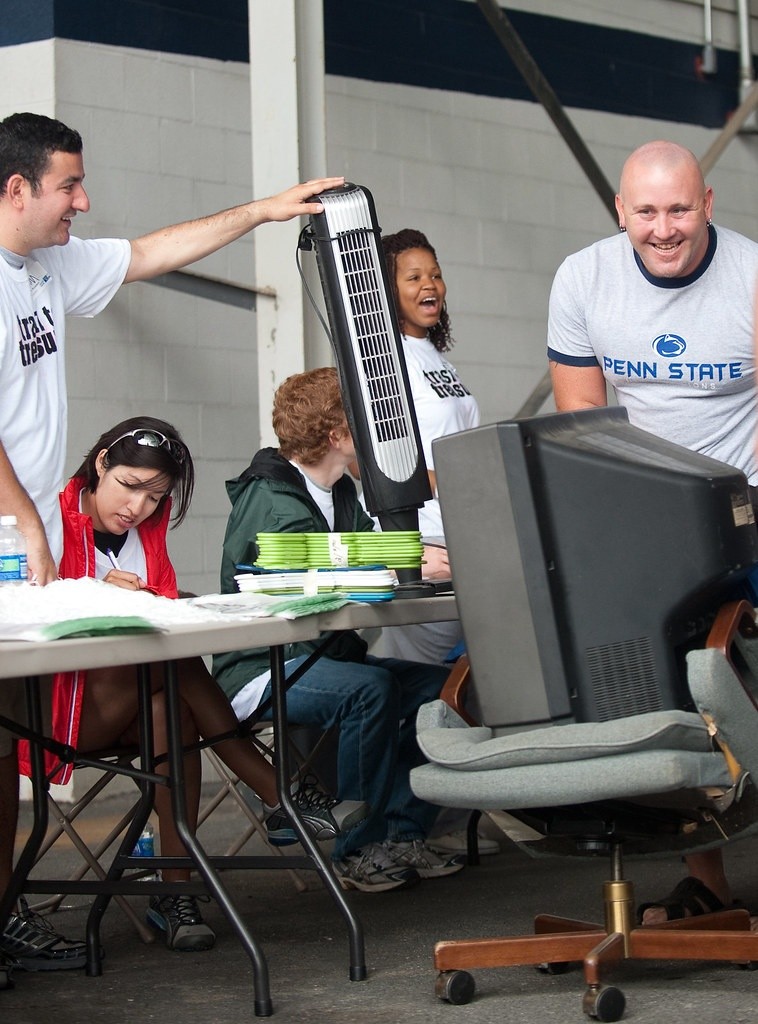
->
[108,427,186,464]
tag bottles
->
[0,515,28,589]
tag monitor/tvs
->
[432,407,757,733]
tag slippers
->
[637,876,745,927]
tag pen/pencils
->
[107,548,122,571]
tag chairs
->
[411,596,758,1022]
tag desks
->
[1,591,466,1014]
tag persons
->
[0,111,347,970]
[547,140,758,971]
[17,416,374,952]
[343,229,499,856]
[212,366,466,895]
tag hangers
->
[233,529,430,605]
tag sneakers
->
[146,882,217,951]
[424,830,500,856]
[0,910,108,970]
[253,762,370,846]
[331,842,422,894]
[382,839,465,879]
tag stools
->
[196,718,311,893]
[10,750,157,947]
